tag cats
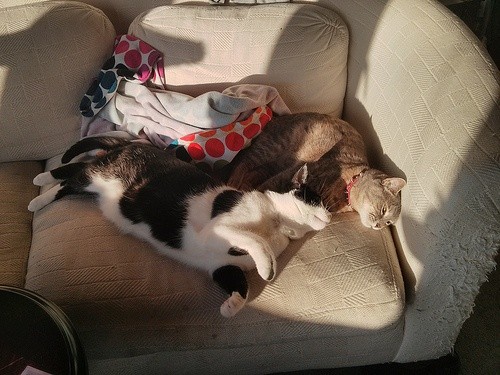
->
[227,112,407,231]
[28,136,340,318]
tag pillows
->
[128,3,350,118]
[1,0,117,162]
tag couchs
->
[0,0,500,375]
